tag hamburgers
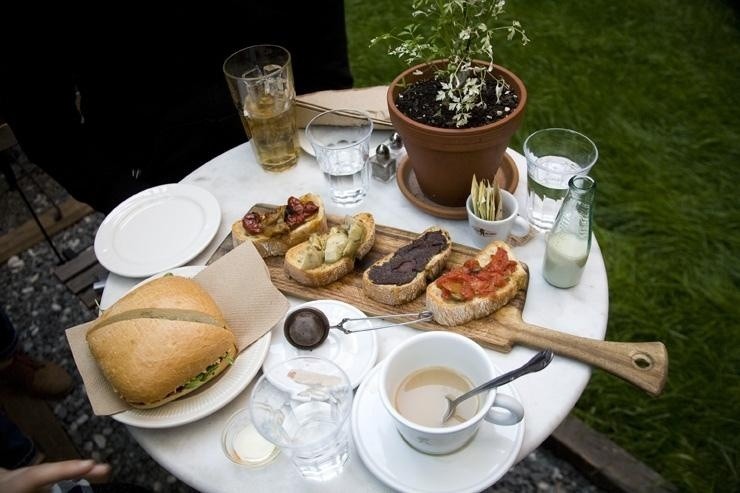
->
[84,274,238,410]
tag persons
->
[0,459,112,493]
[0,307,74,469]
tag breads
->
[425,240,527,326]
[283,213,377,288]
[361,225,453,324]
[231,192,329,259]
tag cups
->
[222,44,302,173]
[378,329,527,454]
[521,127,599,235]
[304,104,373,205]
[249,357,354,484]
[464,185,520,250]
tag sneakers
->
[3,350,74,400]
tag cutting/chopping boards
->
[207,199,671,399]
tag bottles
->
[541,174,598,289]
[368,132,404,179]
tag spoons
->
[439,344,556,424]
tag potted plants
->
[369,0,531,207]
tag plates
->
[222,401,282,467]
[93,181,223,277]
[261,297,377,392]
[351,353,527,493]
[101,265,273,429]
